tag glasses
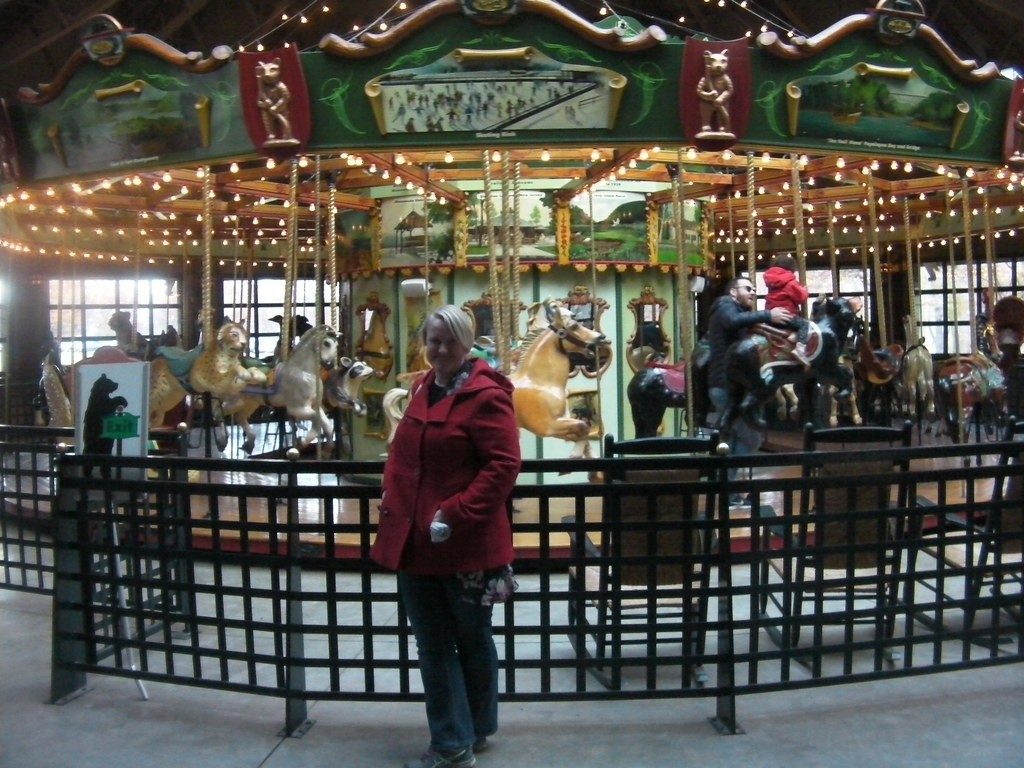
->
[732,285,756,293]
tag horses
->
[208,293,610,484]
[628,292,1010,446]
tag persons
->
[763,255,812,367]
[708,277,795,511]
[369,303,522,768]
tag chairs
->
[897,413,1024,647]
[756,418,920,668]
[565,428,718,692]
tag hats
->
[776,257,795,272]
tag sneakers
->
[728,495,751,510]
[790,347,811,367]
[473,734,487,753]
[405,744,476,768]
